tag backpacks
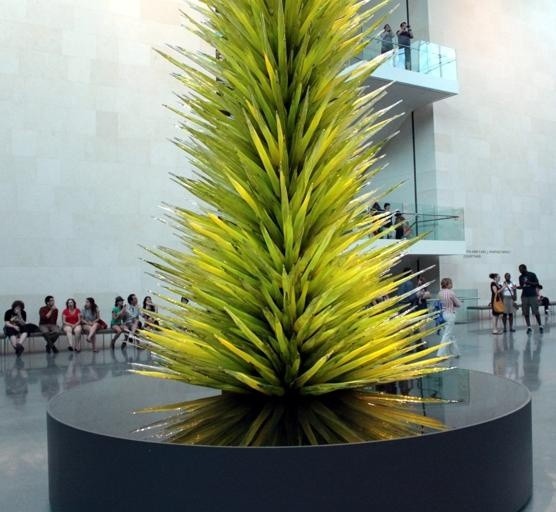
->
[433,300,447,333]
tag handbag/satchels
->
[493,301,506,315]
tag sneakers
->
[10,343,142,354]
[491,326,545,336]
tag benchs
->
[0,326,141,356]
[466,301,556,323]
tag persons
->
[393,265,430,356]
[433,277,463,361]
[37,293,161,352]
[488,272,510,335]
[3,301,28,358]
[491,329,545,393]
[511,263,546,335]
[4,350,155,409]
[370,202,382,239]
[400,216,411,241]
[536,288,551,316]
[381,202,395,239]
[395,20,415,72]
[413,276,432,324]
[379,23,396,56]
[394,209,406,239]
[499,272,519,333]
